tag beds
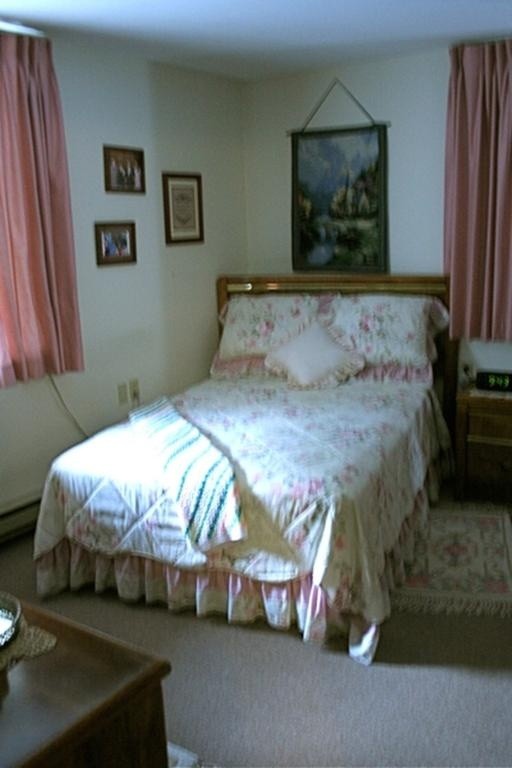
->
[32,270,455,656]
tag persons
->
[111,156,142,189]
[104,239,128,257]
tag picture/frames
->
[290,124,386,275]
[91,145,203,265]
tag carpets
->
[385,501,512,615]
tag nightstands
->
[459,385,511,501]
